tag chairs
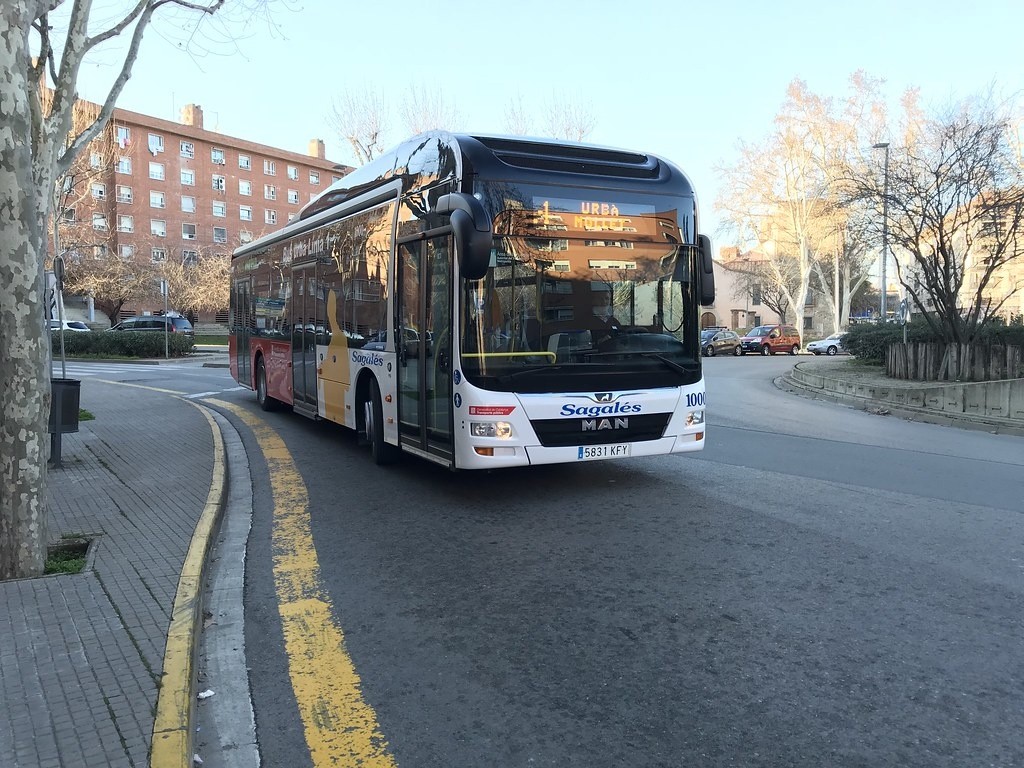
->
[479,313,548,364]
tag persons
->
[582,286,623,344]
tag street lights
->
[873,142,888,323]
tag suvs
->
[741,324,801,356]
[700,326,743,357]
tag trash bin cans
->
[47,377,82,470]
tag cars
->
[44,319,91,332]
[807,331,856,355]
[363,328,435,360]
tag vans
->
[104,315,195,347]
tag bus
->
[226,128,718,469]
